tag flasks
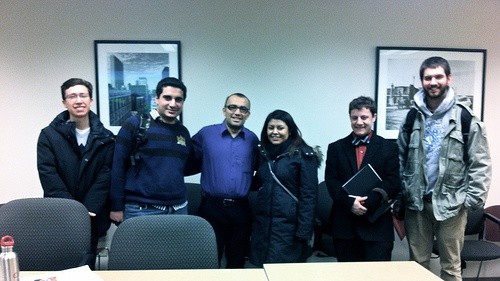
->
[0,235,19,281]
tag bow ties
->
[351,134,370,146]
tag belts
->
[201,191,248,207]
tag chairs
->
[432,204,500,280]
[315,181,337,256]
[107,213,220,269]
[1,199,91,270]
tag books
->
[341,163,383,197]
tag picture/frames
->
[374,45,488,141]
[91,37,182,136]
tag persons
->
[189,93,266,269]
[325,94,401,263]
[37,78,115,271]
[394,55,492,281]
[250,109,318,267]
[109,76,195,222]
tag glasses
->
[65,94,90,99]
[224,104,251,115]
